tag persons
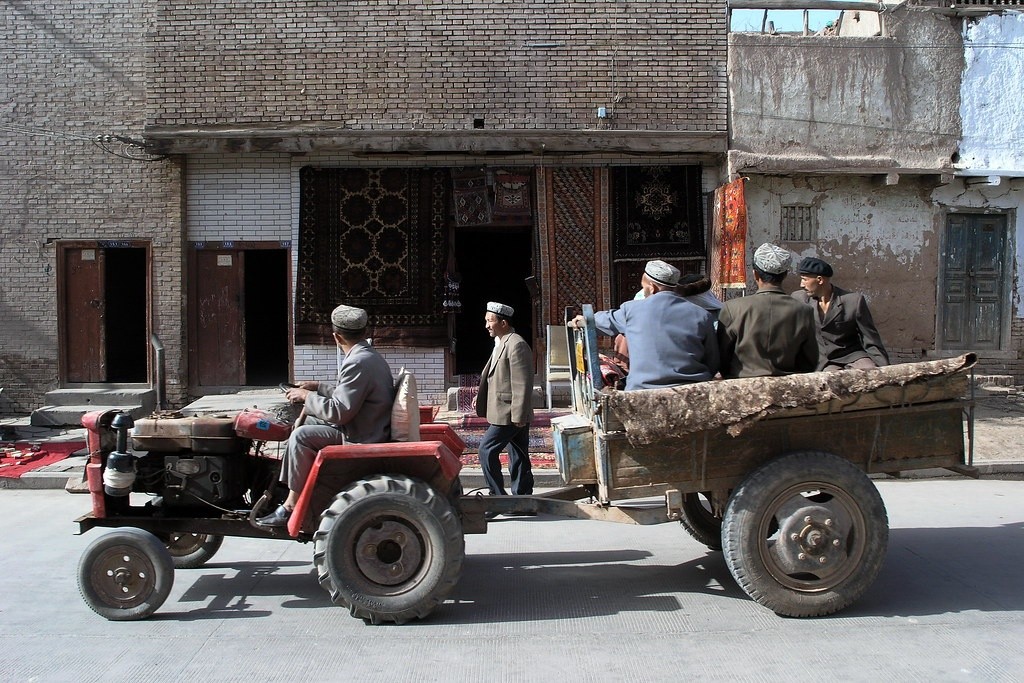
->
[256,304,395,527]
[472,301,537,522]
[572,243,890,392]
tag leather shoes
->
[257,505,292,524]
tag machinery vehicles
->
[65,301,982,624]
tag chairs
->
[543,326,574,409]
[390,366,422,440]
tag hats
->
[754,243,791,275]
[487,301,514,317]
[331,304,368,330]
[795,257,833,277]
[644,260,681,287]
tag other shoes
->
[503,510,537,516]
[485,511,500,519]
